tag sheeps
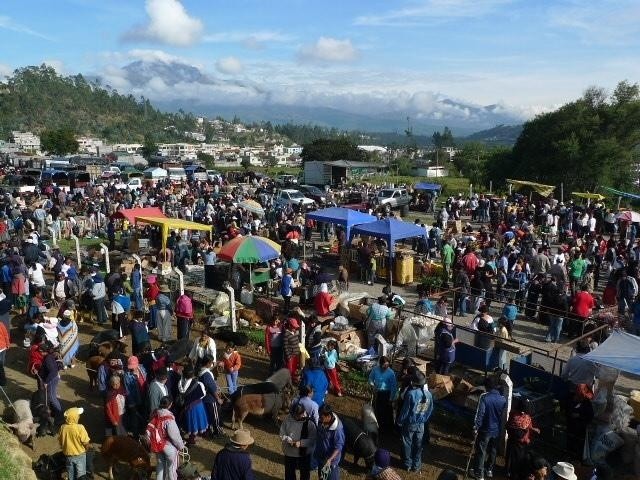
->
[235,309,264,330]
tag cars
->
[100,166,144,191]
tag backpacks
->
[146,414,173,452]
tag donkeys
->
[226,367,293,430]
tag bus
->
[5,165,90,193]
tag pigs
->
[336,414,377,472]
[98,435,156,480]
[89,329,120,356]
[7,399,41,451]
[86,356,106,390]
[97,340,129,356]
[358,401,380,444]
[30,389,56,436]
[33,449,96,480]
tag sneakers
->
[486,471,493,478]
[469,469,484,480]
[188,434,195,443]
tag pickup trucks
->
[379,187,413,213]
[276,189,314,211]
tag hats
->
[552,462,578,480]
[320,283,328,292]
[27,239,33,244]
[478,305,490,314]
[289,402,305,415]
[209,198,214,202]
[183,366,194,374]
[77,408,84,414]
[127,356,139,369]
[158,285,172,293]
[63,311,72,320]
[39,306,49,313]
[478,259,486,267]
[374,449,390,468]
[287,268,292,274]
[301,263,307,269]
[146,276,156,284]
[232,216,238,220]
[298,213,302,215]
[288,318,299,329]
[410,371,426,385]
[230,429,254,445]
[65,300,76,310]
[35,344,50,352]
[443,318,454,326]
[88,267,98,274]
[57,272,65,277]
[176,461,198,480]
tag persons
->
[0,155,639,480]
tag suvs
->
[301,184,332,207]
